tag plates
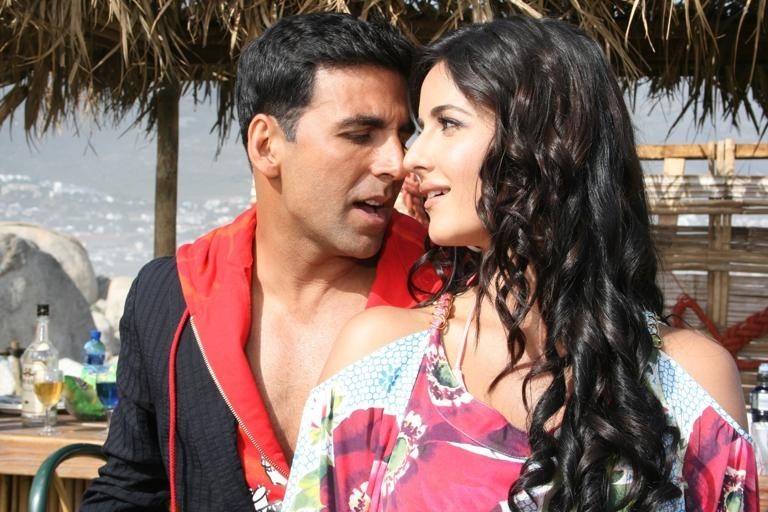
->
[0,398,70,416]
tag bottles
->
[18,303,60,428]
[748,362,768,476]
[82,329,106,377]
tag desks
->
[1,413,111,510]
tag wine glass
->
[32,369,66,438]
[95,370,120,438]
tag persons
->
[76,13,486,511]
[276,15,759,511]
